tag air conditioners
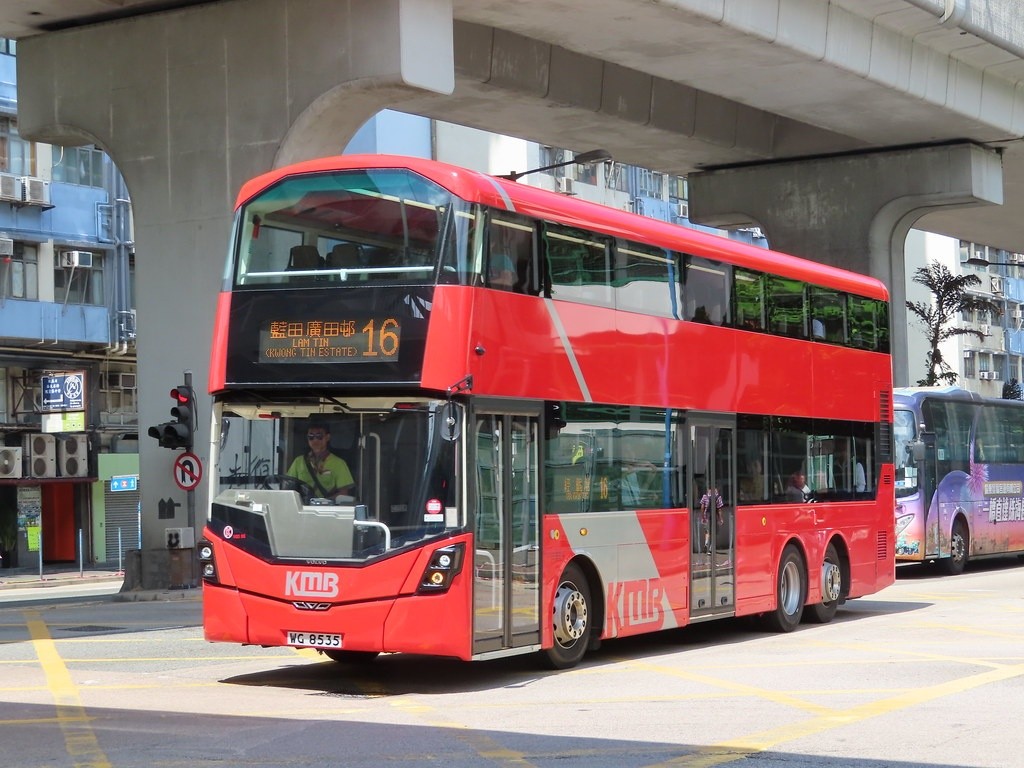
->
[23,433,56,478]
[0,172,21,201]
[106,373,136,390]
[61,251,92,268]
[58,435,89,478]
[0,238,14,256]
[0,447,22,479]
[21,176,51,205]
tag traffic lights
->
[166,386,194,448]
[148,421,178,450]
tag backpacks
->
[706,493,721,513]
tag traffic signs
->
[111,477,137,492]
[40,373,86,412]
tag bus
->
[806,435,875,493]
[198,157,893,678]
[893,385,1024,574]
[556,423,697,470]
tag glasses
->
[306,432,324,440]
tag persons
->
[699,481,724,555]
[620,440,658,506]
[785,469,810,493]
[738,456,764,500]
[284,422,356,499]
[799,308,824,339]
[457,230,515,286]
[830,437,866,492]
